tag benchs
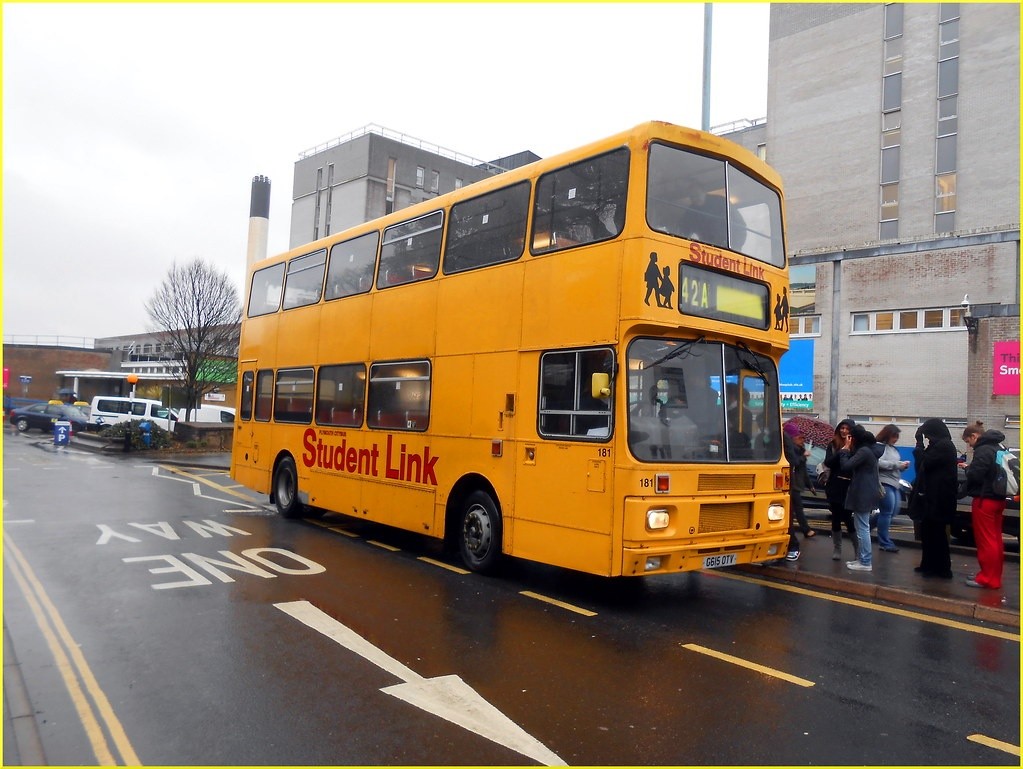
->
[257,397,428,429]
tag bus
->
[230,118,794,579]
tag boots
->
[847,529,859,560]
[831,530,842,560]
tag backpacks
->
[984,444,1020,496]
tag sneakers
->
[786,550,800,561]
[846,559,872,571]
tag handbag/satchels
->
[815,440,835,487]
[877,479,887,499]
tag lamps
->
[960,293,978,334]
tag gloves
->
[915,426,924,448]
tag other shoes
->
[966,576,986,588]
[914,566,953,579]
[879,544,899,552]
[804,530,816,538]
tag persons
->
[647,376,752,463]
[824,419,886,571]
[755,412,818,562]
[958,420,1009,589]
[679,182,747,253]
[868,424,909,553]
[905,418,959,580]
[576,367,615,436]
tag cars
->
[10,399,89,434]
[66,401,92,418]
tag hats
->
[784,423,799,438]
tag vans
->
[177,404,236,423]
[86,395,180,437]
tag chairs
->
[503,241,535,259]
[273,265,445,310]
[553,231,582,248]
